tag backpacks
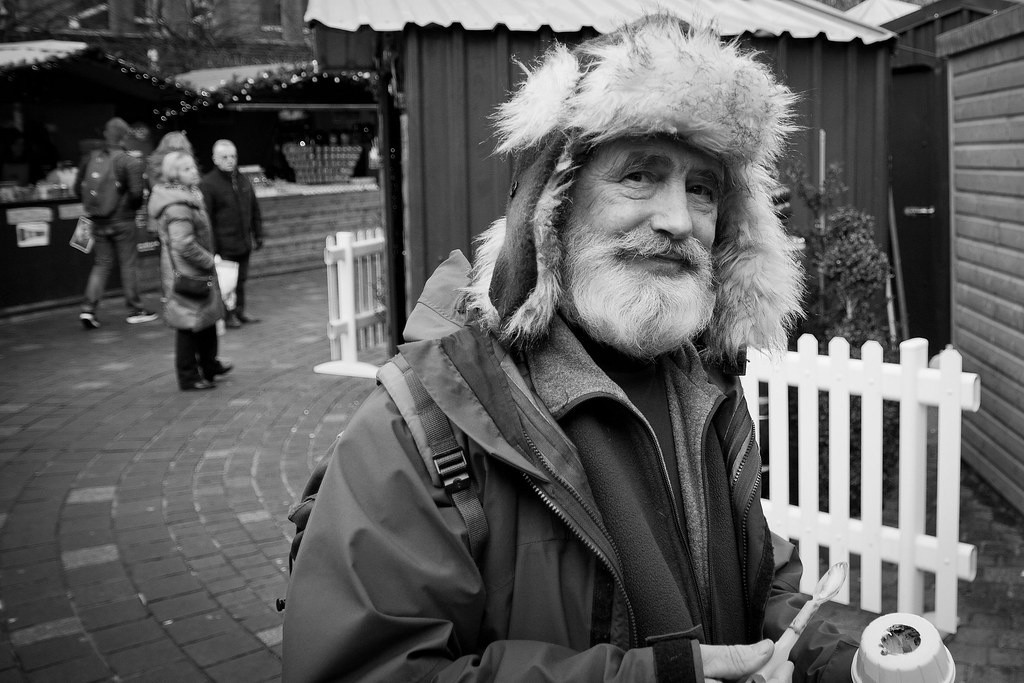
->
[275,351,492,610]
[80,149,129,218]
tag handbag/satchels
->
[215,255,239,310]
[172,272,212,302]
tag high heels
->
[180,376,216,390]
[202,360,233,382]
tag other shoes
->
[238,316,263,322]
[225,315,241,327]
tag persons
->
[278,23,893,683]
[74,117,265,390]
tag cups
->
[851,613,956,683]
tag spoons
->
[750,561,848,683]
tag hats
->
[107,118,134,134]
[462,13,805,354]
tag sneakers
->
[79,305,101,328]
[126,307,159,323]
